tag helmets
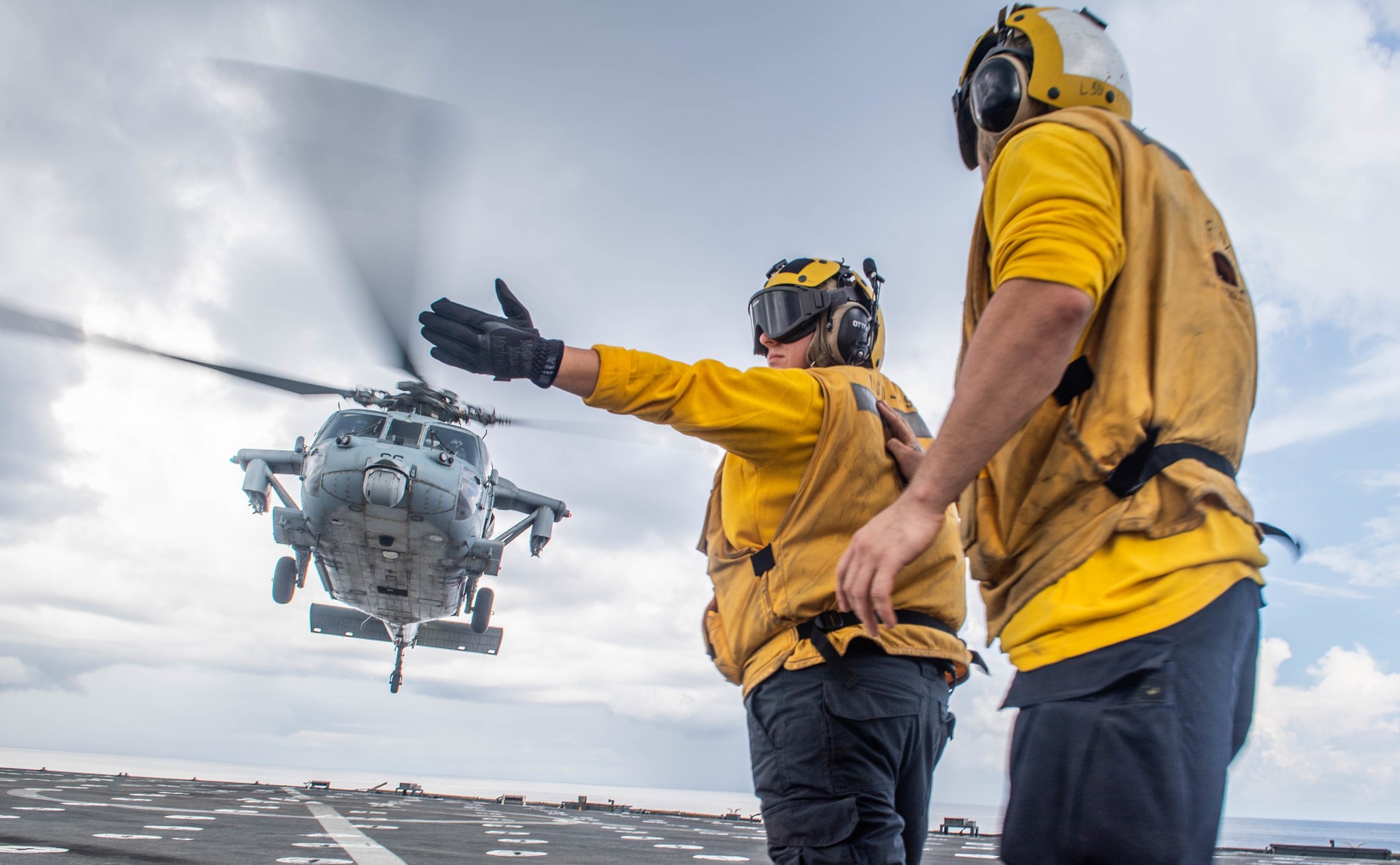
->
[761,256,886,373]
[955,2,1134,124]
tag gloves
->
[417,277,565,389]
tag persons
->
[834,0,1304,863]
[433,437,466,460]
[337,418,374,438]
[417,257,989,865]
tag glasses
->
[951,77,981,172]
[747,285,866,357]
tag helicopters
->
[1,61,726,695]
